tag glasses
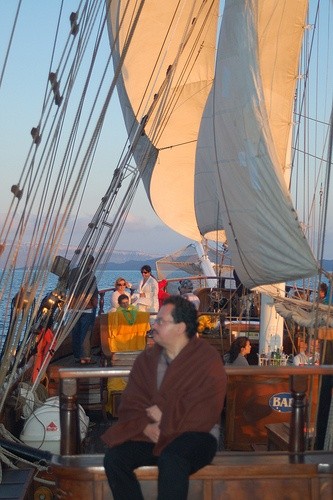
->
[142,272,149,274]
[116,284,126,286]
[149,319,179,326]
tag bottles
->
[260,346,281,367]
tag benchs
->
[0,439,333,500]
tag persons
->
[60,255,98,365]
[226,337,252,367]
[293,271,333,314]
[292,334,320,367]
[109,264,201,313]
[103,296,229,500]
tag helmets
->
[179,280,194,292]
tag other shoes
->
[81,358,98,364]
